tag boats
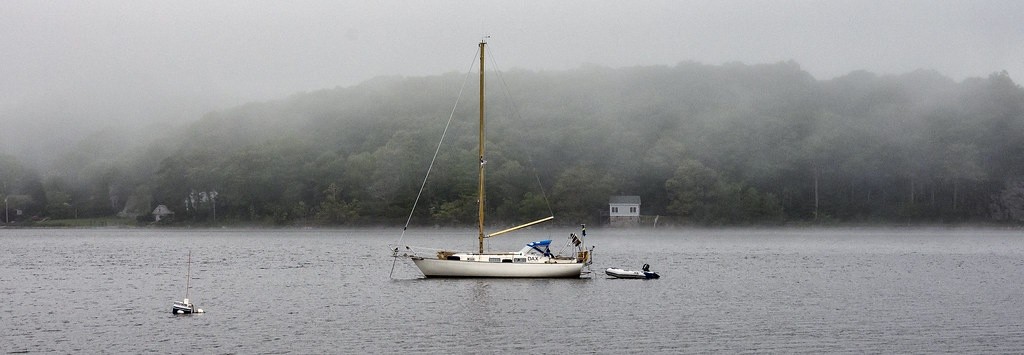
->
[605,266,660,279]
[172,249,206,315]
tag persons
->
[546,246,550,257]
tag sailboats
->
[387,34,596,278]
